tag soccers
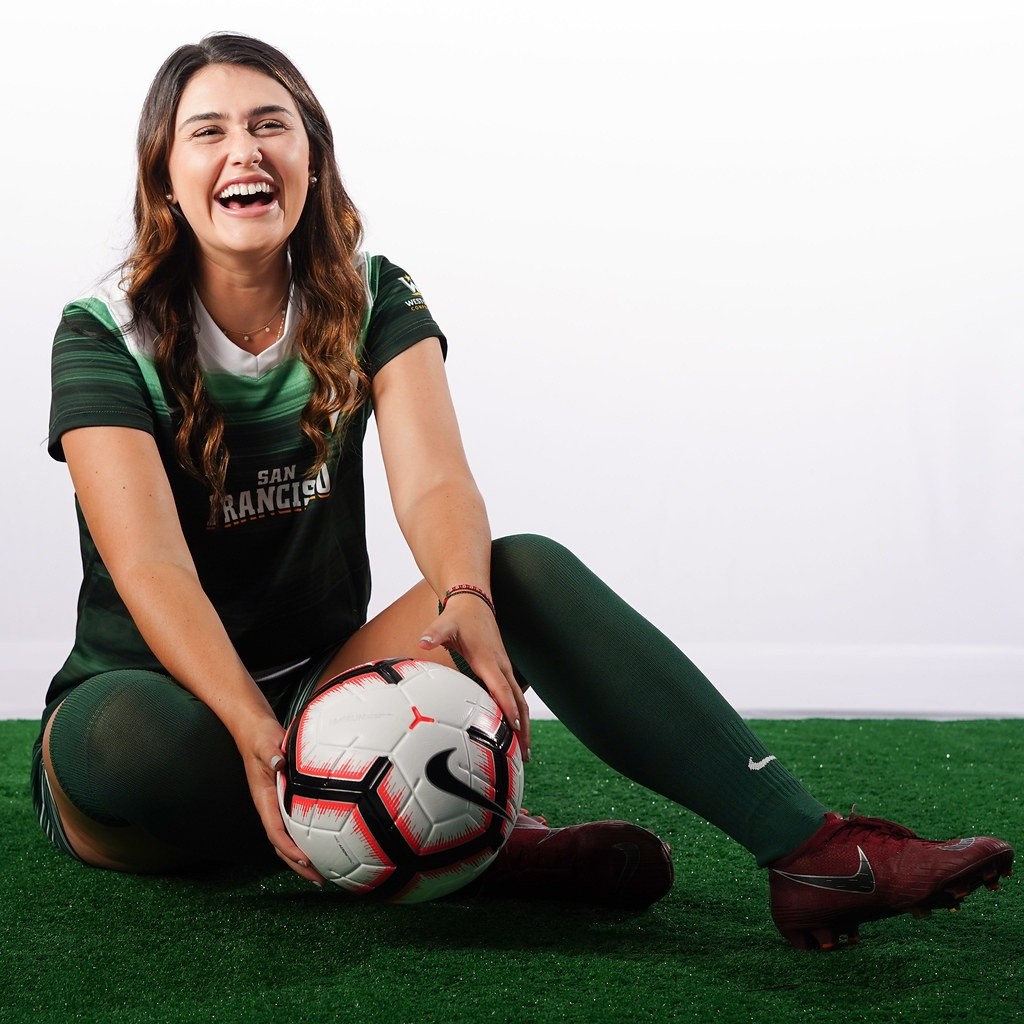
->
[273,655,530,907]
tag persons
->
[35,30,1010,955]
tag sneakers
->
[419,804,675,928]
[770,806,1012,953]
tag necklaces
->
[213,304,286,342]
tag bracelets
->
[443,584,496,619]
